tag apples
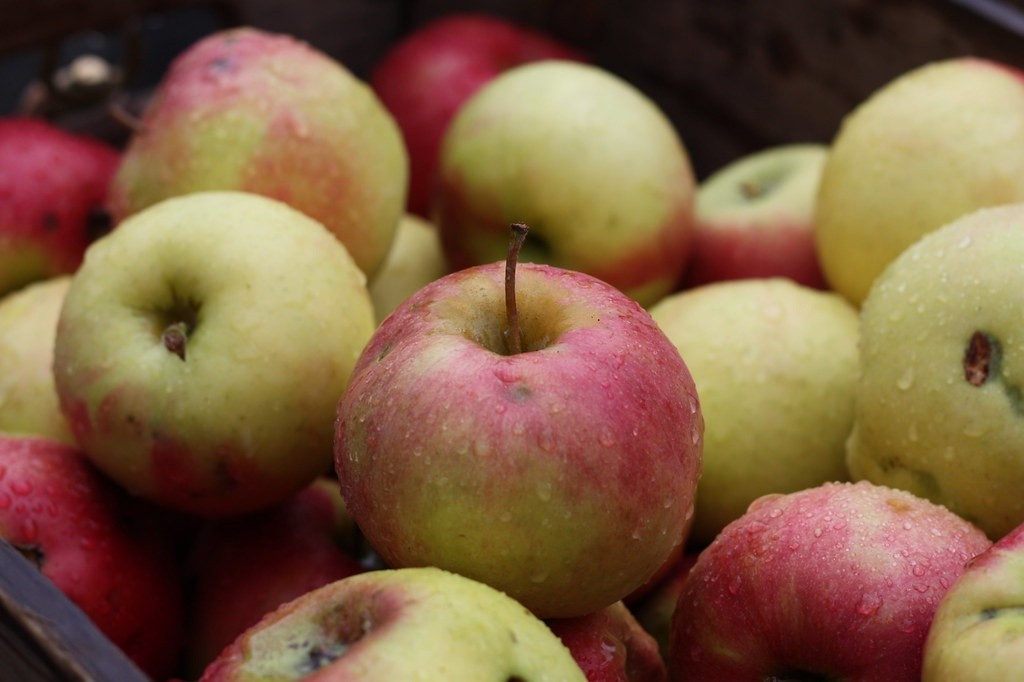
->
[0,15,1024,682]
